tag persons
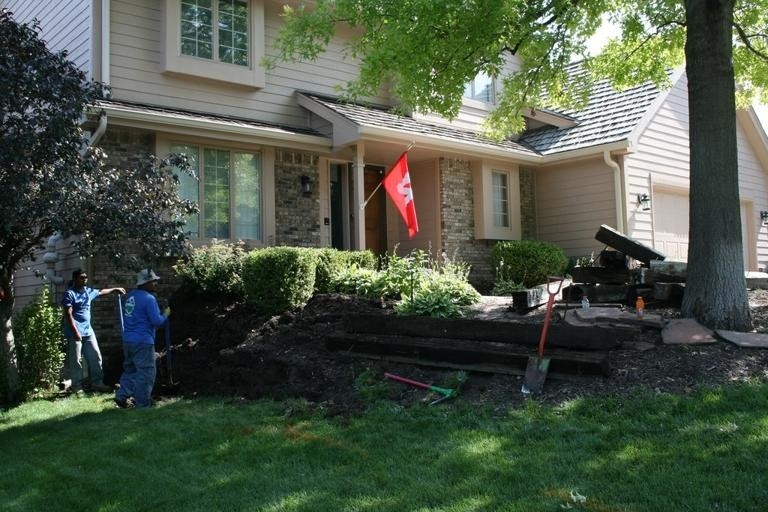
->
[61,268,125,396]
[112,266,172,410]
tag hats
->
[136,267,161,286]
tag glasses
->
[77,275,88,280]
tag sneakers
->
[116,395,134,408]
[136,400,156,408]
[73,388,85,398]
[91,384,111,392]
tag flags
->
[383,153,419,241]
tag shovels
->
[524,274,563,392]
[160,298,180,396]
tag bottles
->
[635,296,645,320]
[581,296,590,314]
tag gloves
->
[163,306,171,316]
